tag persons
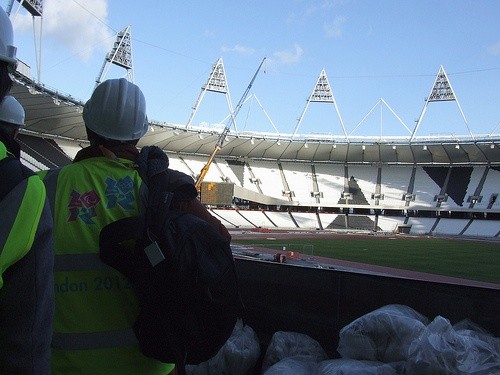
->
[35,78,200,375]
[0,58,55,375]
[0,96,25,163]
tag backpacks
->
[99,213,239,363]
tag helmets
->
[0,5,17,63]
[82,78,148,140]
[0,95,25,126]
